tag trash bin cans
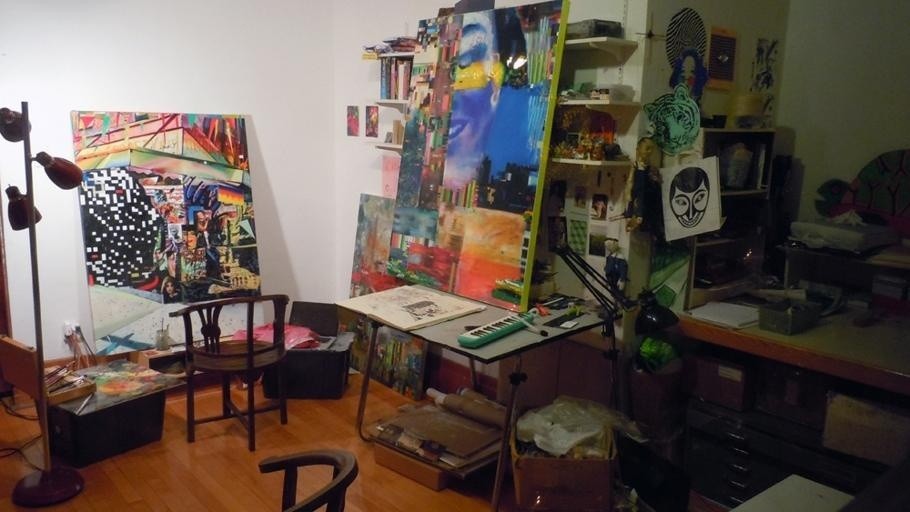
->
[508,405,618,512]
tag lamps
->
[1,96,92,509]
[553,235,684,342]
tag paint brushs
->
[511,314,549,336]
[44,360,87,394]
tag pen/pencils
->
[75,392,94,416]
[161,318,169,335]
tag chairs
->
[163,290,303,449]
[251,446,358,510]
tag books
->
[362,41,415,147]
[334,282,487,332]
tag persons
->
[153,205,257,303]
[625,137,661,233]
[443,7,529,191]
[591,197,607,220]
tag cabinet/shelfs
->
[372,49,414,155]
[550,36,643,169]
[667,114,779,314]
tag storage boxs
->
[685,349,752,415]
[820,382,910,466]
[498,412,621,510]
[749,354,831,428]
[264,300,355,402]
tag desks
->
[327,273,626,511]
[660,240,910,512]
